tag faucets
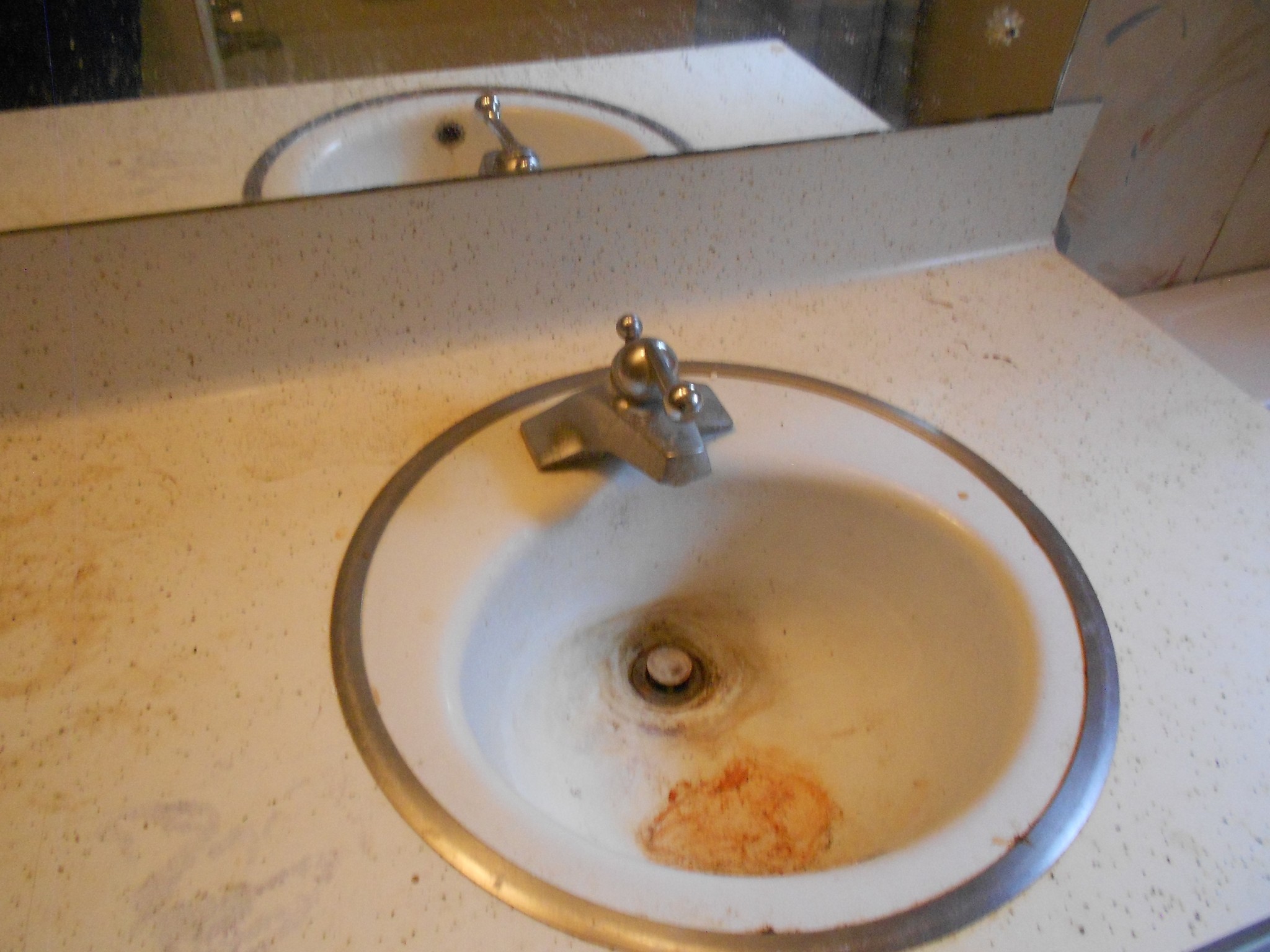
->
[473,94,540,176]
[520,314,734,487]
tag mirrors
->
[0,0,1093,238]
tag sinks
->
[329,359,1118,952]
[247,86,689,206]
